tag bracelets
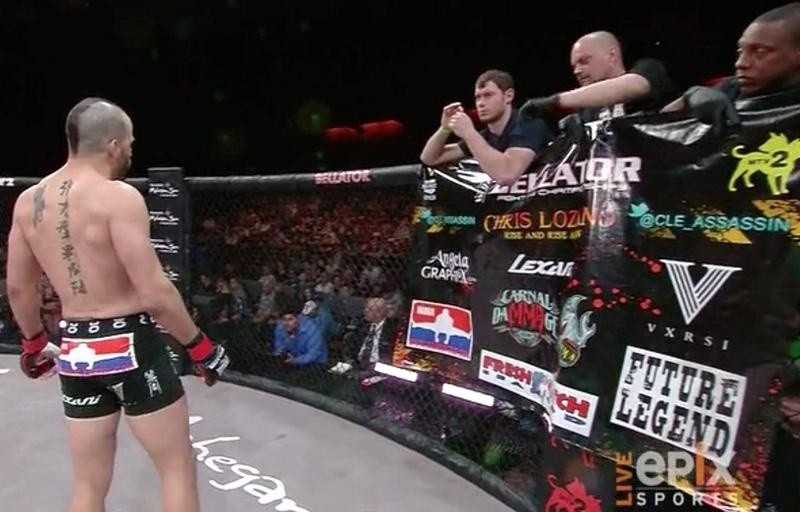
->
[440,126,450,137]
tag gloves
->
[560,114,583,134]
[185,329,231,386]
[517,94,559,123]
[685,86,742,136]
[20,332,62,382]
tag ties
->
[361,325,376,371]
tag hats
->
[303,300,317,314]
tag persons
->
[187,184,420,403]
[1,97,231,512]
[520,30,652,122]
[420,69,551,187]
[0,231,61,347]
[658,4,799,131]
